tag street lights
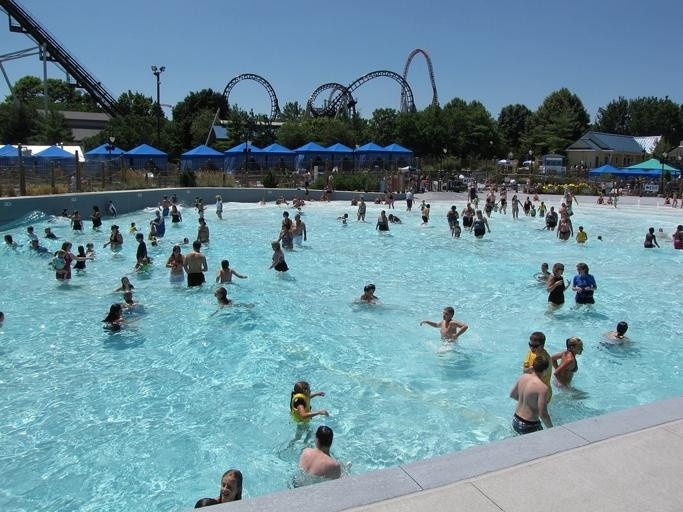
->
[237,129,255,172]
[439,147,447,170]
[150,65,164,148]
[659,151,667,194]
[105,135,115,185]
[676,151,683,197]
[528,150,533,174]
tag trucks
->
[521,158,566,174]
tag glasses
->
[528,341,543,348]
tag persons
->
[608,321,628,339]
[420,307,468,341]
[299,425,342,479]
[541,263,598,306]
[271,180,682,249]
[552,337,583,387]
[359,284,378,301]
[269,241,290,272]
[508,356,553,436]
[5,194,248,322]
[523,332,552,404]
[292,381,329,445]
[217,468,244,504]
[195,498,217,508]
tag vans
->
[499,160,514,173]
